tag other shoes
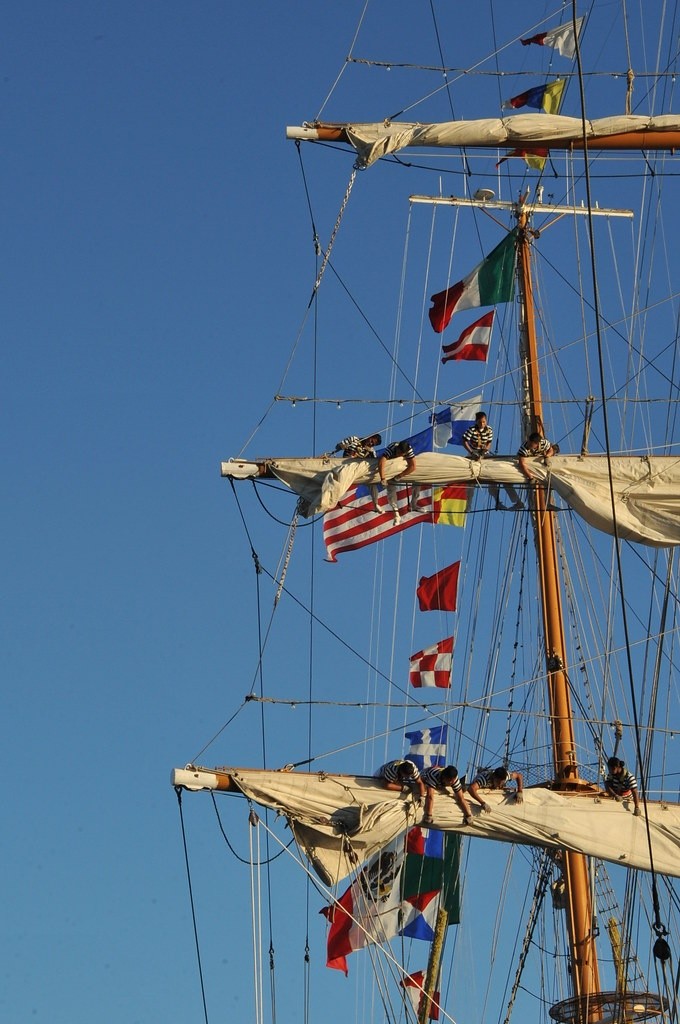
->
[496,502,507,510]
[376,505,385,514]
[465,507,470,512]
[393,516,401,526]
[548,504,561,511]
[409,506,426,513]
[506,503,524,511]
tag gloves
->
[380,477,400,488]
[482,802,492,814]
[529,478,535,488]
[633,808,641,816]
[471,449,489,459]
[514,792,524,804]
[403,785,410,792]
[543,457,552,467]
[465,815,473,827]
[615,795,623,802]
[419,796,426,807]
[423,816,434,825]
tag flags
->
[318,826,460,1020]
[322,395,482,564]
[403,725,448,769]
[407,636,455,688]
[495,17,585,170]
[441,310,494,364]
[428,226,519,333]
[416,561,460,611]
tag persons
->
[603,758,641,816]
[335,433,385,514]
[373,759,523,826]
[463,411,562,512]
[379,441,427,527]
